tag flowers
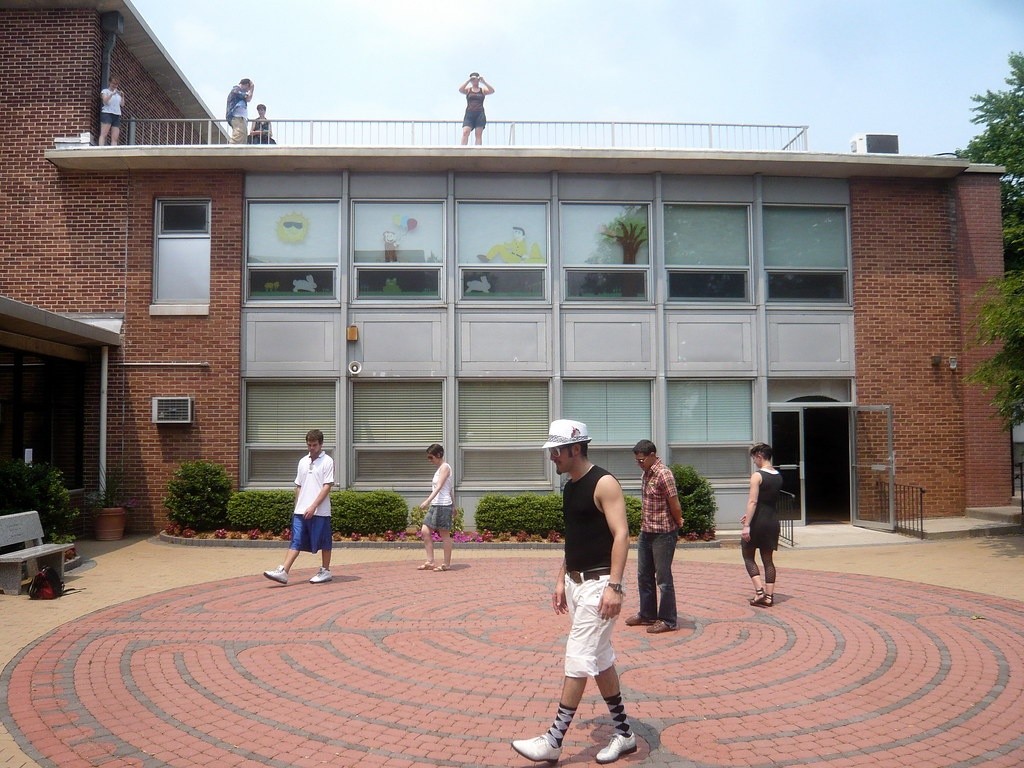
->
[86,460,139,520]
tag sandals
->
[762,594,773,606]
[750,587,766,605]
[417,562,433,570]
[433,564,450,571]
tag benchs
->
[0,510,75,596]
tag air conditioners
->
[848,133,900,154]
[151,396,196,424]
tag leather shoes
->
[511,735,562,765]
[596,731,637,763]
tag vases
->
[90,507,127,542]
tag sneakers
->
[647,620,674,633]
[624,612,655,625]
[263,565,288,584]
[309,567,332,583]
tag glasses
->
[636,454,650,463]
[549,444,573,457]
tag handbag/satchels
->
[29,565,64,599]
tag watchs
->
[608,583,621,592]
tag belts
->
[567,567,610,583]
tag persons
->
[251,104,272,144]
[264,429,334,584]
[625,439,685,633]
[458,72,494,145]
[227,79,254,144]
[99,76,125,146]
[739,442,783,607]
[510,419,637,766]
[417,444,457,572]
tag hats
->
[542,420,592,448]
